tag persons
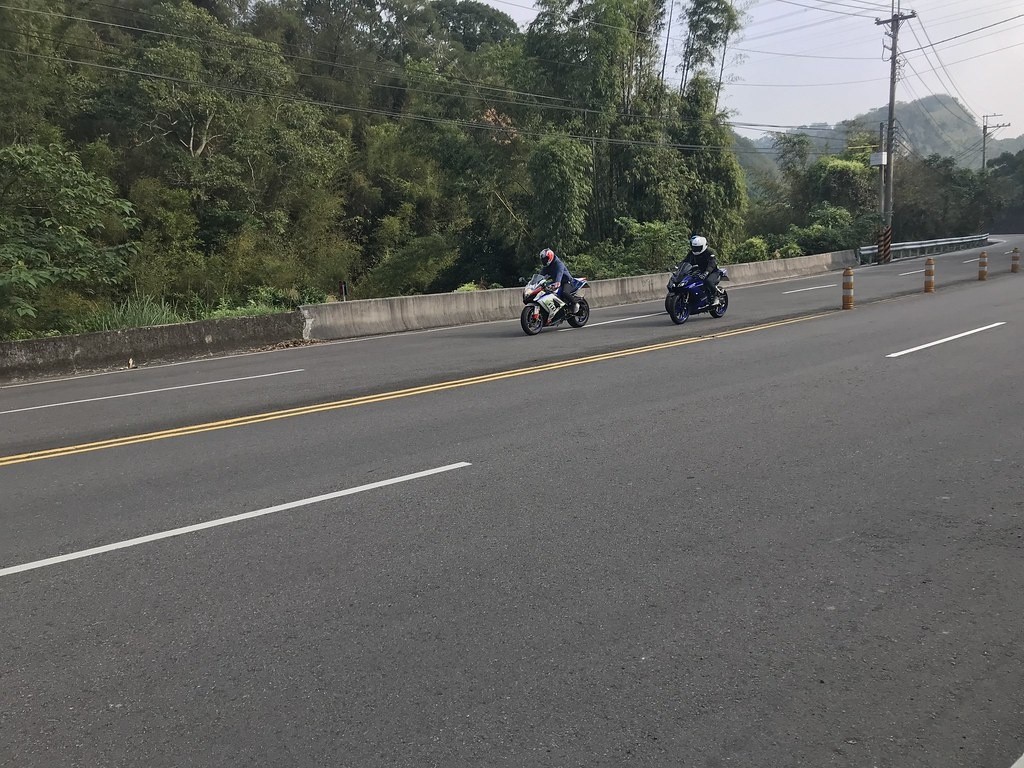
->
[684,236,722,306]
[538,248,581,314]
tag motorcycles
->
[665,263,730,325]
[519,274,590,335]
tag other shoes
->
[711,297,721,306]
[573,303,580,313]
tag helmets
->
[690,236,699,246]
[540,249,554,267]
[692,237,707,255]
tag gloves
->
[699,271,709,280]
